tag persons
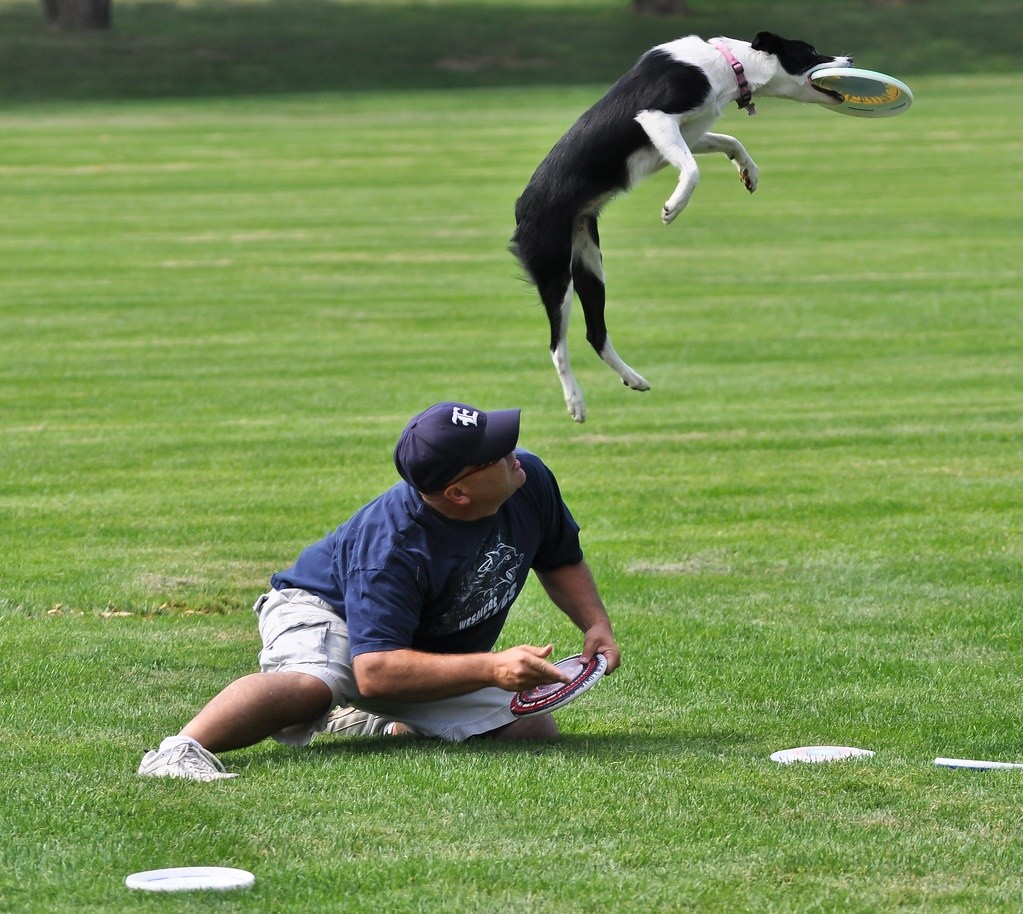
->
[131,401,621,781]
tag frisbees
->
[812,67,914,119]
[510,651,608,719]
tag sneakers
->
[138,734,240,782]
[318,704,388,743]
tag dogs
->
[507,32,852,424]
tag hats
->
[393,402,523,491]
